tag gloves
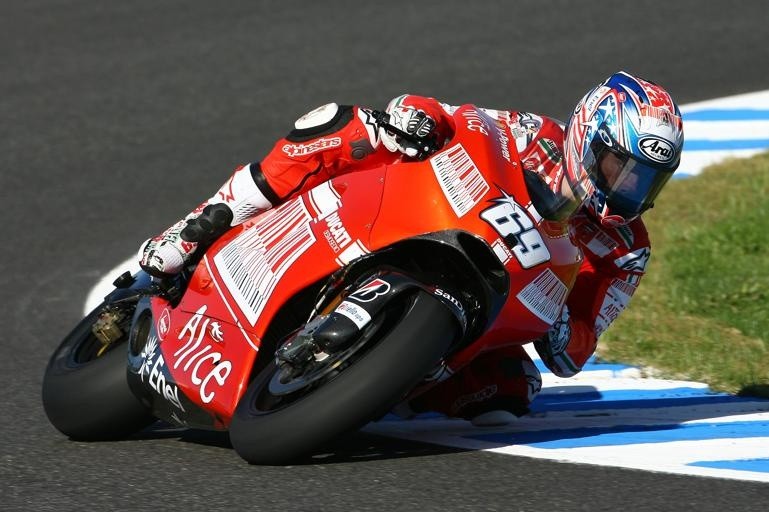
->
[376,104,441,159]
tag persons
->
[137,68,685,427]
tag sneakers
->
[136,215,203,277]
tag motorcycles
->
[38,95,603,470]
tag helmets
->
[556,68,688,230]
